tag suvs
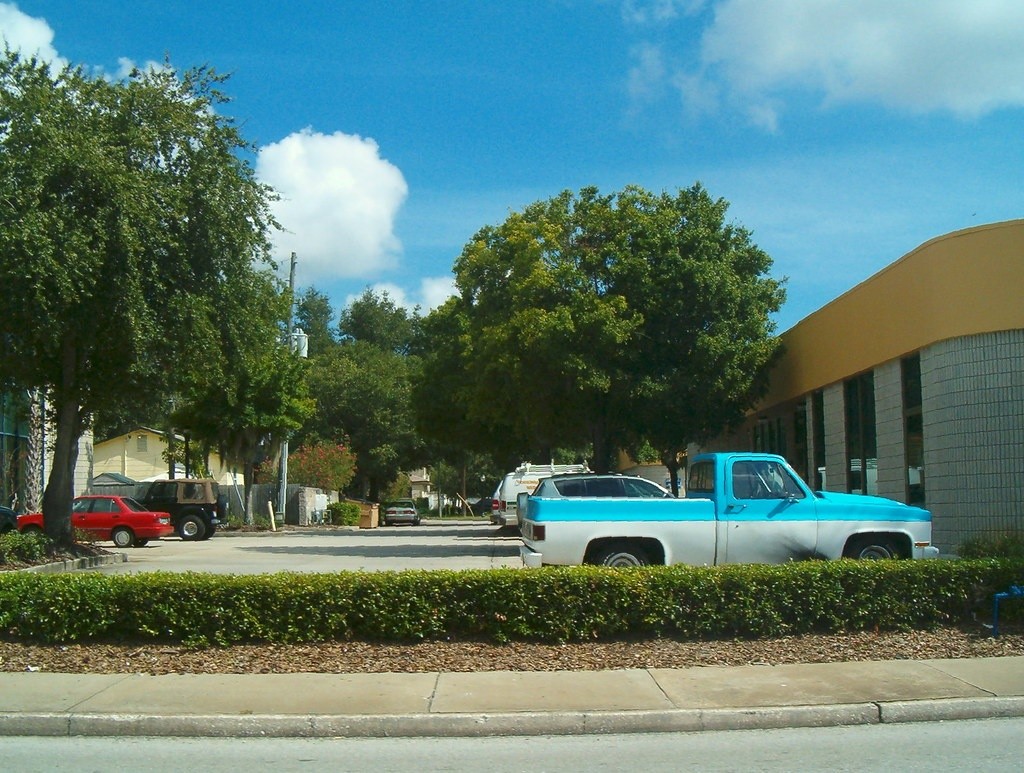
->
[140,479,228,541]
[517,472,675,531]
[497,458,593,538]
[490,479,503,525]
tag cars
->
[385,500,419,526]
[18,496,175,549]
[0,506,18,535]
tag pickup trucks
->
[521,451,939,568]
